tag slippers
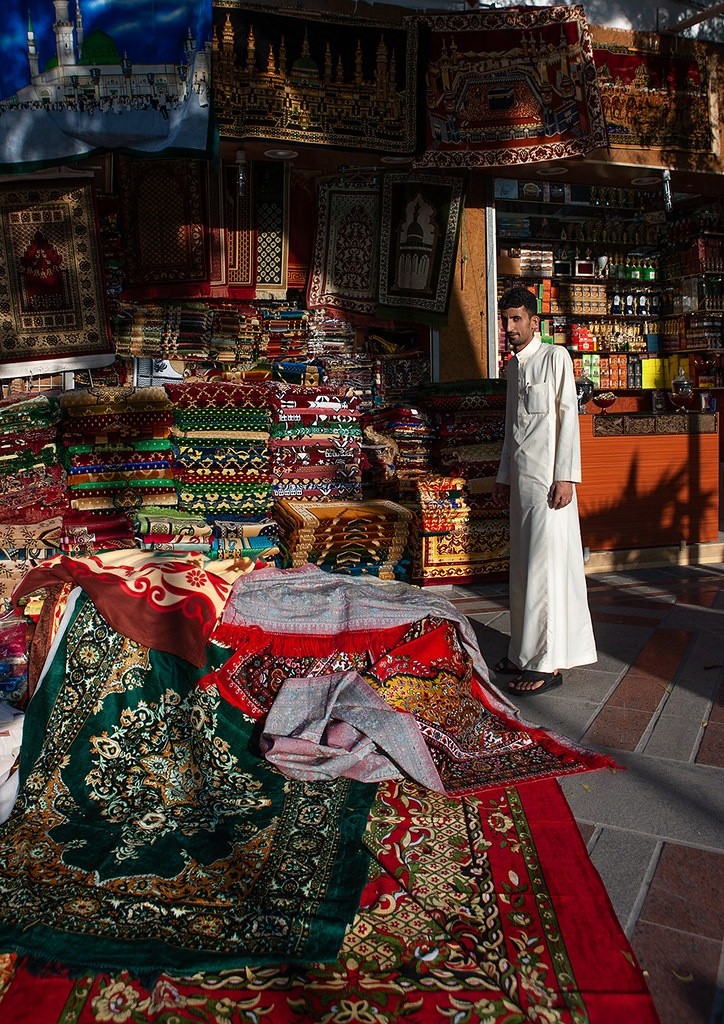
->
[494,655,564,696]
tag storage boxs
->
[641,358,666,389]
[619,368,627,380]
[618,354,628,368]
[497,316,609,390]
[664,352,723,388]
[628,363,634,376]
[526,279,661,316]
[607,354,618,368]
[629,356,639,364]
[628,376,635,389]
[636,363,641,377]
[610,369,618,380]
[635,377,641,388]
[685,313,722,349]
[610,380,619,390]
[649,353,659,358]
[639,353,648,359]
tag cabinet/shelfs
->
[662,232,724,390]
[492,198,664,392]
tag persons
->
[492,286,581,700]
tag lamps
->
[235,146,247,197]
